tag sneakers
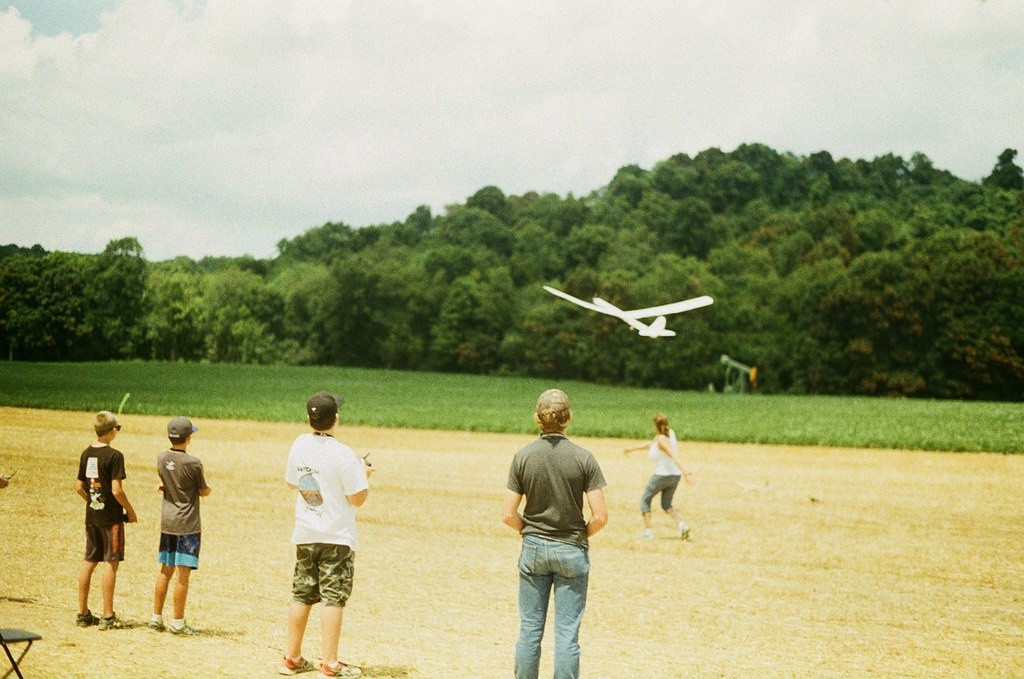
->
[169,626,200,637]
[76,612,100,628]
[147,620,168,634]
[98,617,127,631]
[317,661,362,679]
[278,654,319,675]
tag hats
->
[168,416,198,439]
[536,389,570,424]
[306,394,345,421]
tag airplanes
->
[543,284,714,340]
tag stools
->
[0,628,41,679]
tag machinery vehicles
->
[720,354,757,394]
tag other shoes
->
[680,523,690,541]
[642,528,654,537]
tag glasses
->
[109,425,121,431]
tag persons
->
[280,393,376,679]
[503,389,609,679]
[76,410,136,630]
[146,418,211,636]
[625,415,692,540]
